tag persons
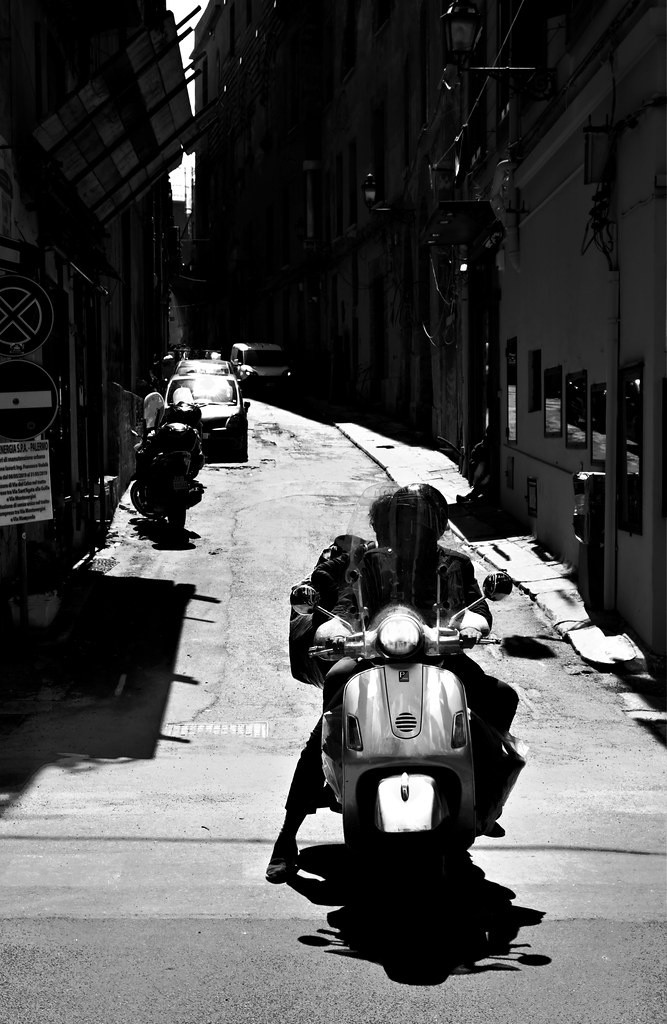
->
[267,488,519,884]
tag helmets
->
[388,483,448,542]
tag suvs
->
[230,342,293,387]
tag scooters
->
[287,563,511,882]
[131,409,197,533]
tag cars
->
[161,359,251,440]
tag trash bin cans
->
[574,472,606,615]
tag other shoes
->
[486,823,505,838]
[265,840,298,879]
